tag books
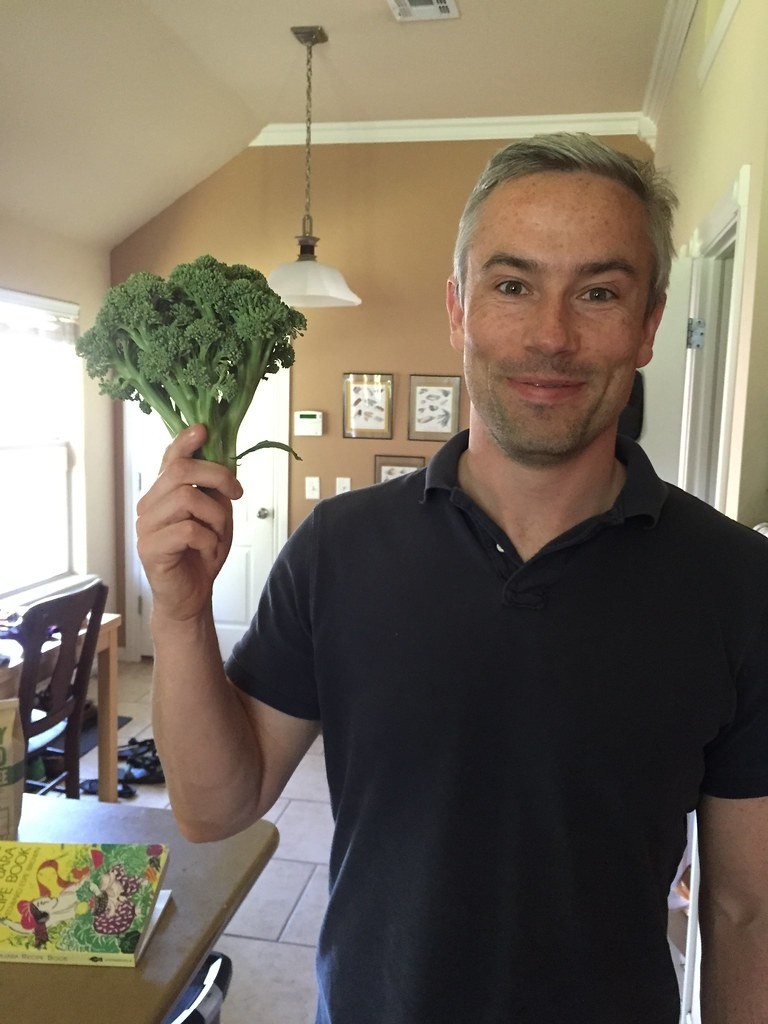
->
[0,840,174,968]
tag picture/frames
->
[375,455,424,486]
[343,373,394,441]
[409,376,459,442]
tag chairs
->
[22,582,111,805]
[159,948,232,1024]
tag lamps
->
[262,23,365,321]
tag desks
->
[0,789,347,1024]
[0,611,124,807]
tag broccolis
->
[72,256,304,516]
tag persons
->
[136,131,767,1024]
